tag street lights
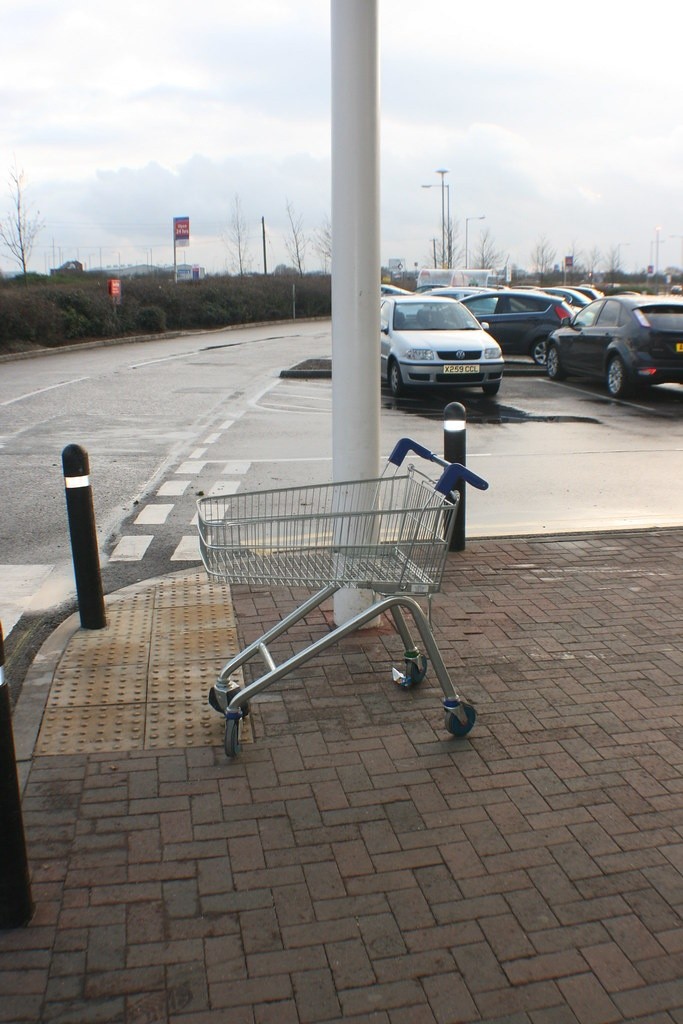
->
[436,170,449,270]
[421,184,451,269]
[465,217,485,269]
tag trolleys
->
[195,437,490,757]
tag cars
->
[380,294,506,397]
[381,284,605,316]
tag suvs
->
[447,291,576,364]
[546,294,683,397]
